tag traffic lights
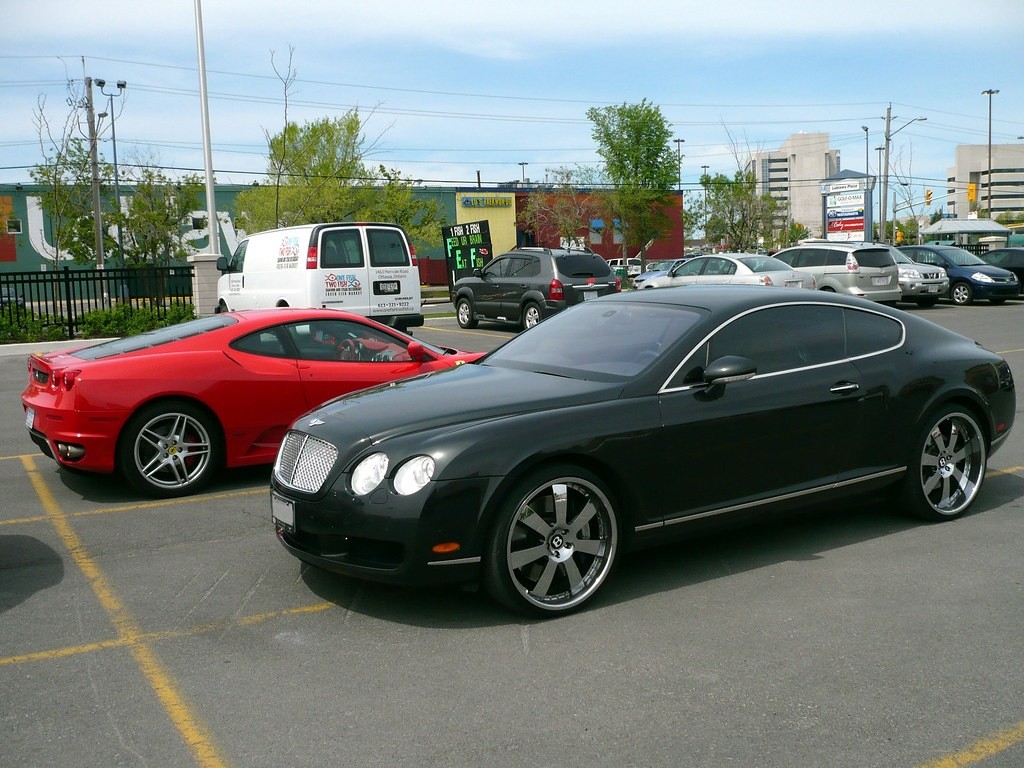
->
[896,232,904,242]
[925,190,933,205]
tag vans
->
[216,223,426,340]
[605,258,641,278]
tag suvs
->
[798,240,951,309]
[753,245,902,307]
[450,245,620,332]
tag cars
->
[897,244,1019,304]
[0,287,25,310]
[632,258,708,288]
[269,287,1017,620]
[685,243,739,258]
[642,254,817,299]
[977,247,1024,294]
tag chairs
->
[708,263,719,275]
[640,316,696,365]
[326,246,341,264]
[514,267,531,277]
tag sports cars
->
[22,306,492,497]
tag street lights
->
[882,116,926,243]
[518,161,528,182]
[702,165,709,244]
[674,139,686,190]
[861,126,869,242]
[94,78,127,309]
[982,89,1000,219]
[876,146,886,243]
